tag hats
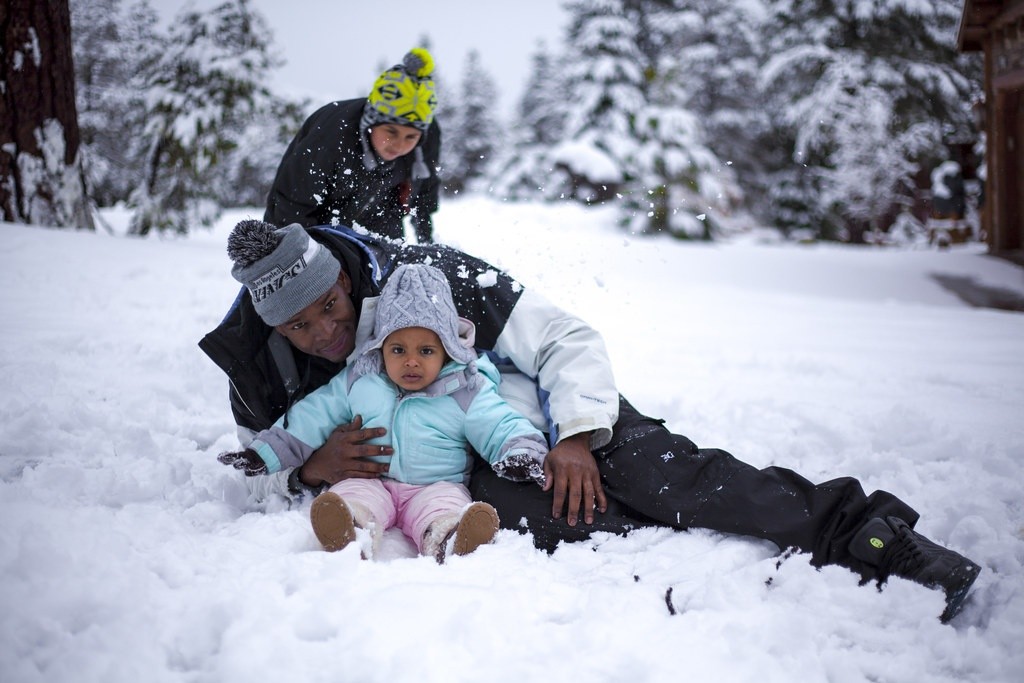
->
[227,219,341,327]
[359,47,438,180]
[353,264,476,391]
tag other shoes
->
[436,502,500,565]
[310,492,365,562]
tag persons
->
[261,46,441,244]
[217,266,551,564]
[198,217,985,627]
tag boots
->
[848,513,981,621]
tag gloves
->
[493,454,546,487]
[216,449,268,477]
